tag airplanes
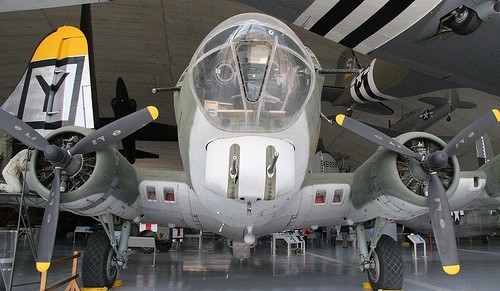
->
[233,0,500,142]
[0,12,500,291]
[93,76,178,165]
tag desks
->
[117,237,156,268]
